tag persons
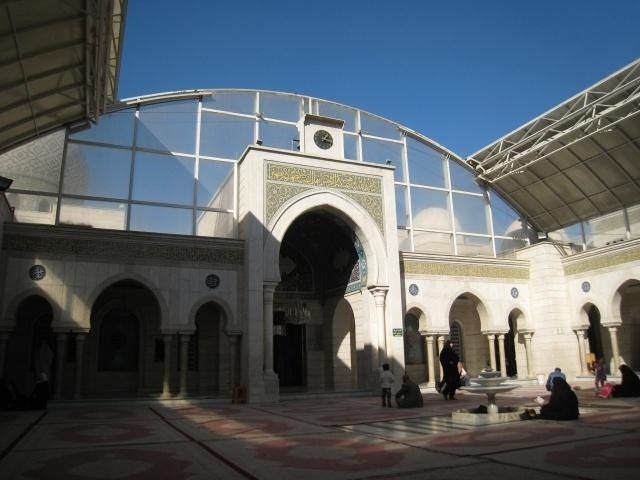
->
[435,340,470,401]
[395,375,424,408]
[478,361,491,378]
[546,367,567,391]
[379,364,395,408]
[540,377,580,421]
[612,365,639,398]
[0,368,50,411]
[592,357,608,391]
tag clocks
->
[314,130,334,149]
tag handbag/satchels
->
[456,361,466,379]
